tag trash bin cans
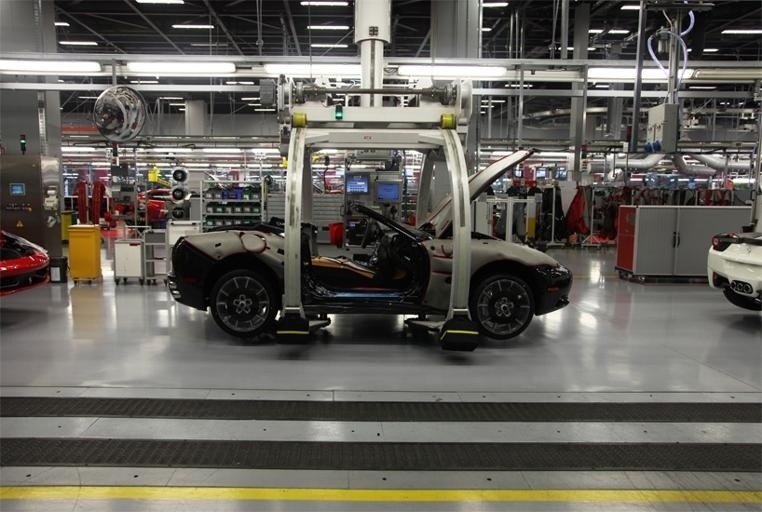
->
[49,257,67,283]
[67,224,101,285]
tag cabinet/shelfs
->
[200,176,268,231]
[615,205,753,284]
[143,228,169,287]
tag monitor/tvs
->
[373,179,402,206]
[8,182,26,197]
[536,167,547,180]
[346,174,369,194]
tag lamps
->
[262,62,363,76]
[397,64,508,78]
[126,61,236,73]
[1,58,102,73]
[587,67,696,79]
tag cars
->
[707,222,762,312]
[0,229,51,298]
